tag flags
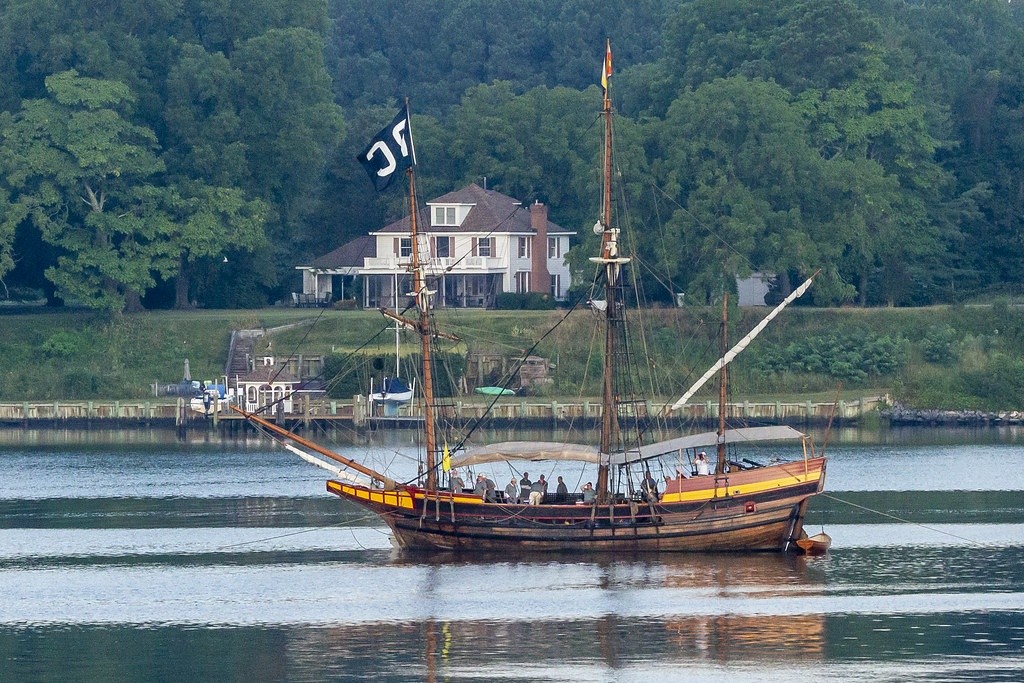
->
[357,104,412,192]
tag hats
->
[698,452,706,455]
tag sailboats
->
[367,252,416,405]
[228,38,839,553]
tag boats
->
[795,532,833,557]
[190,383,233,414]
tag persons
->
[693,452,709,476]
[557,476,568,504]
[580,482,595,504]
[448,470,549,504]
[641,471,658,502]
